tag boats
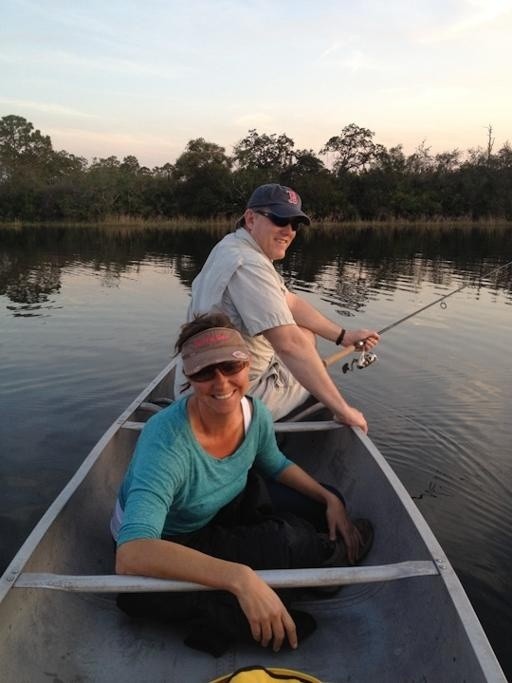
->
[1,354,507,683]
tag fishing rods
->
[324,263,510,367]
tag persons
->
[173,184,380,436]
[108,312,375,657]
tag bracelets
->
[336,328,346,346]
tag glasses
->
[256,209,299,230]
[182,360,246,382]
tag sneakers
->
[230,609,316,645]
[308,518,374,599]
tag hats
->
[246,183,311,226]
[181,327,250,376]
